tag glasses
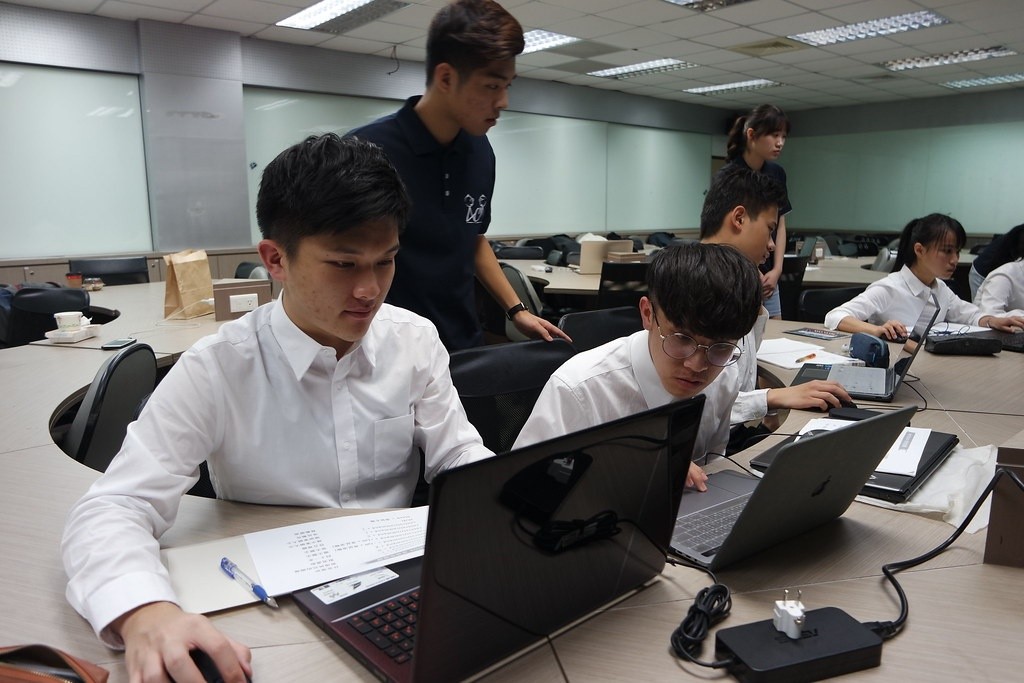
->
[650,300,746,367]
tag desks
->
[0,249,1024,683]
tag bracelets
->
[987,317,993,327]
[505,302,529,321]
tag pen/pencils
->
[934,331,952,334]
[795,354,816,363]
[221,557,279,609]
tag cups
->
[53,311,83,333]
[65,271,82,288]
[796,242,803,254]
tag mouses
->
[166,648,252,683]
[806,397,857,415]
[880,331,909,344]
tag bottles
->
[815,242,825,259]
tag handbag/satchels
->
[0,282,90,349]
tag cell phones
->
[102,338,137,350]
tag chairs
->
[451,230,675,455]
[63,342,159,475]
[235,262,270,280]
[778,222,1024,330]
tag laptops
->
[668,404,921,571]
[799,236,819,257]
[972,328,1024,353]
[790,294,940,403]
[287,394,708,683]
[748,416,960,504]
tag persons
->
[344,0,572,343]
[968,225,1024,317]
[727,102,792,318]
[63,133,496,683]
[700,173,852,456]
[511,242,763,492]
[825,213,1024,340]
